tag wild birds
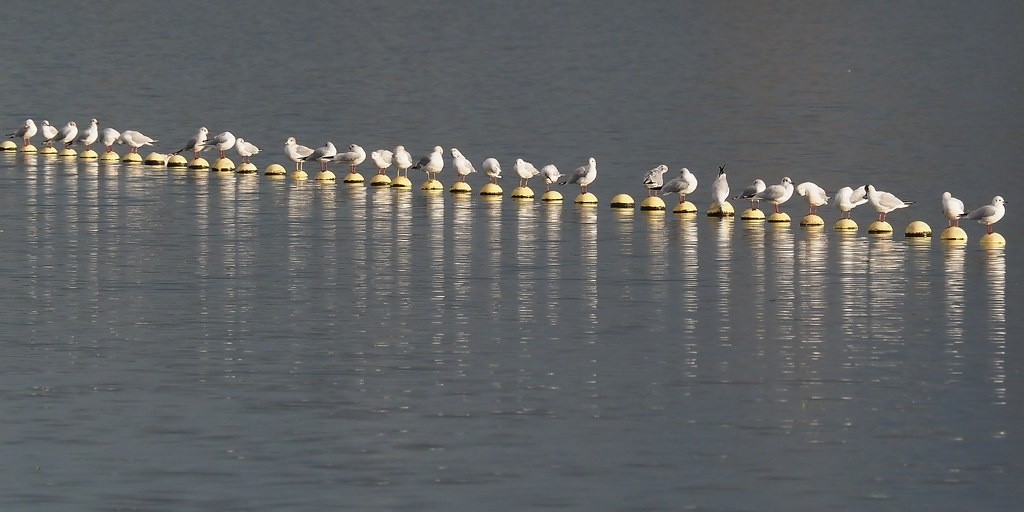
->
[942,192,968,226]
[5,117,159,153]
[235,138,262,162]
[482,158,503,183]
[412,145,444,179]
[450,148,478,182]
[284,137,315,171]
[514,159,541,188]
[835,184,916,221]
[796,181,831,215]
[711,165,734,214]
[661,168,698,204]
[732,176,795,213]
[558,157,597,194]
[371,145,412,177]
[641,164,668,197]
[297,142,366,173]
[957,195,1008,234]
[173,126,236,159]
[541,164,562,191]
